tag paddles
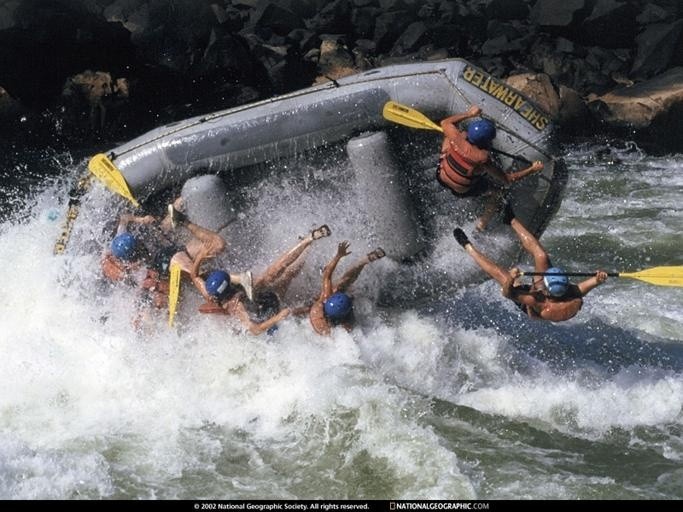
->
[516,265,683,287]
[383,101,542,167]
[88,154,182,251]
[167,261,182,329]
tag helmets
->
[543,267,568,297]
[111,232,137,260]
[324,293,351,320]
[206,271,230,297]
[467,119,496,144]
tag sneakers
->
[167,203,189,231]
[502,200,515,224]
[453,227,473,249]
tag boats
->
[92,56,567,329]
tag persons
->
[434,104,545,200]
[189,223,332,337]
[308,239,386,339]
[99,196,226,344]
[450,201,608,323]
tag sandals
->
[310,224,332,240]
[366,247,386,262]
